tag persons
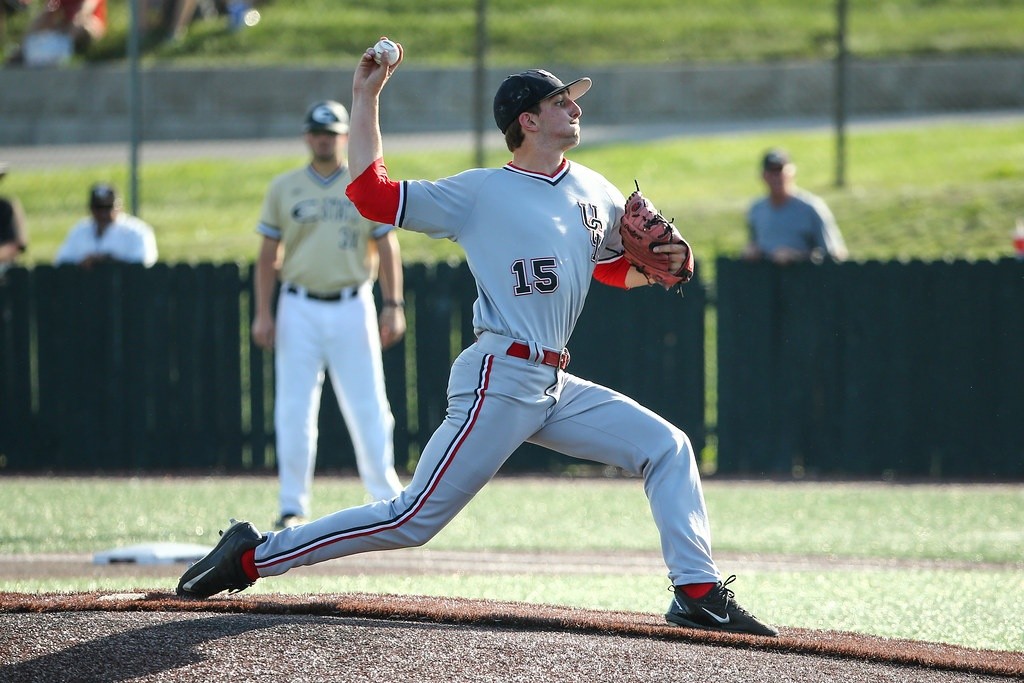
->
[747,150,847,261]
[177,35,778,637]
[0,162,27,281]
[251,100,408,531]
[8,0,266,67]
[53,184,159,269]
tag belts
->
[286,288,358,303]
[505,343,572,371]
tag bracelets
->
[381,298,406,308]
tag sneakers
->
[666,575,780,637]
[175,519,261,598]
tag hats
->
[90,186,115,208]
[302,100,349,134]
[763,150,792,171]
[494,70,593,134]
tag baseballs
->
[373,40,400,67]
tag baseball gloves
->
[618,189,695,293]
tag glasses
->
[310,129,341,137]
